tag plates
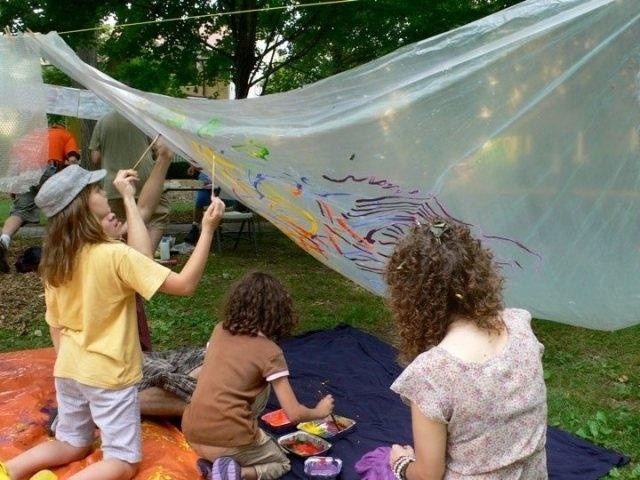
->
[297,414,356,439]
[303,456,343,479]
[261,408,292,430]
[277,430,333,458]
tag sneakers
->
[197,457,241,480]
[0,238,10,272]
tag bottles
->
[160,239,170,260]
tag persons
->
[181,276,335,480]
[89,107,172,257]
[99,136,175,374]
[0,113,82,273]
[185,165,221,244]
[355,216,549,479]
[0,164,226,480]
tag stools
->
[213,210,262,254]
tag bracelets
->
[391,457,416,480]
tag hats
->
[34,164,108,217]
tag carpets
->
[263,320,631,480]
[5,341,199,478]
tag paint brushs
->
[318,390,340,431]
[132,133,161,170]
[211,153,215,196]
